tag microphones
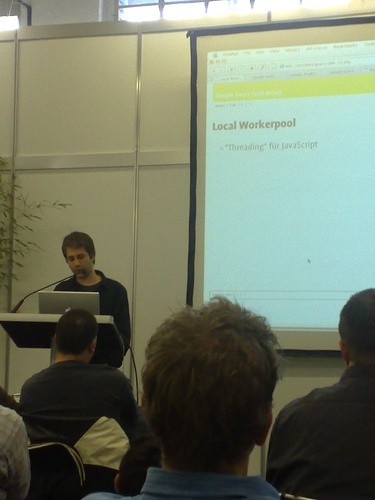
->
[11,270,89,313]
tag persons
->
[53,232,131,369]
[80,295,280,500]
[19,308,138,500]
[0,386,31,500]
[265,289,375,500]
[114,440,163,497]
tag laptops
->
[38,291,101,315]
